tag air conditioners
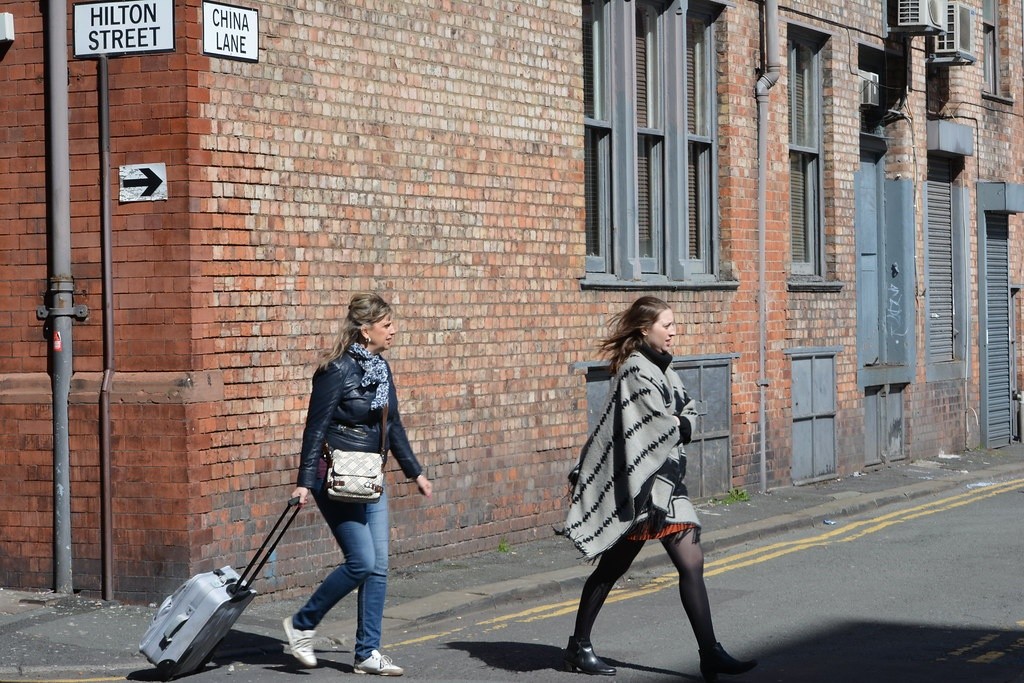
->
[934,2,976,63]
[898,0,948,32]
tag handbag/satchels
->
[326,449,385,503]
[568,462,581,486]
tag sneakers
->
[354,650,403,675]
[283,616,317,666]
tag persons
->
[555,296,759,683]
[283,292,431,677]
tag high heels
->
[562,635,617,675]
[698,643,757,683]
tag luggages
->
[138,496,301,682]
